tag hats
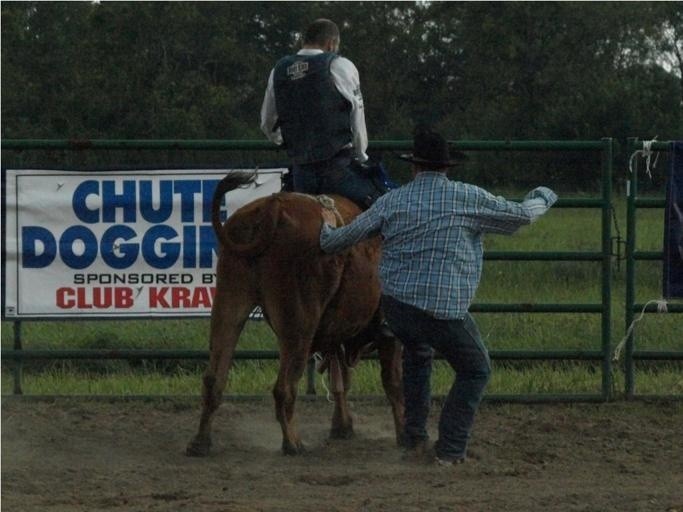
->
[399,131,464,168]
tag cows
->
[186,170,406,457]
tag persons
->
[321,128,561,469]
[256,18,395,214]
